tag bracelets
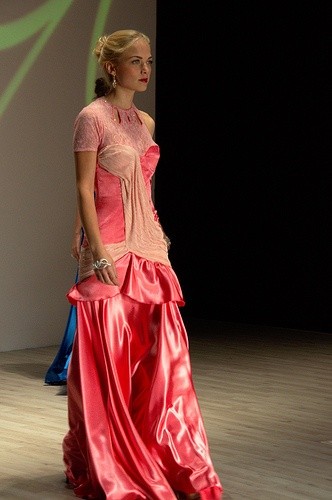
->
[92,258,111,270]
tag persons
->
[60,30,226,500]
[42,76,118,384]
